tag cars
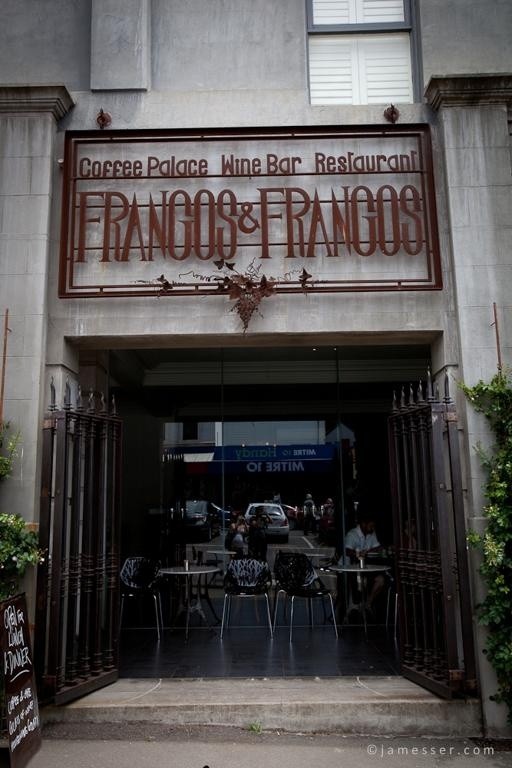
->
[177,500,220,544]
[241,502,290,543]
[278,504,303,531]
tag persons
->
[250,506,274,562]
[225,513,248,560]
[336,514,381,619]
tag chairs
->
[114,547,422,645]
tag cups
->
[184,560,190,571]
[358,558,366,569]
